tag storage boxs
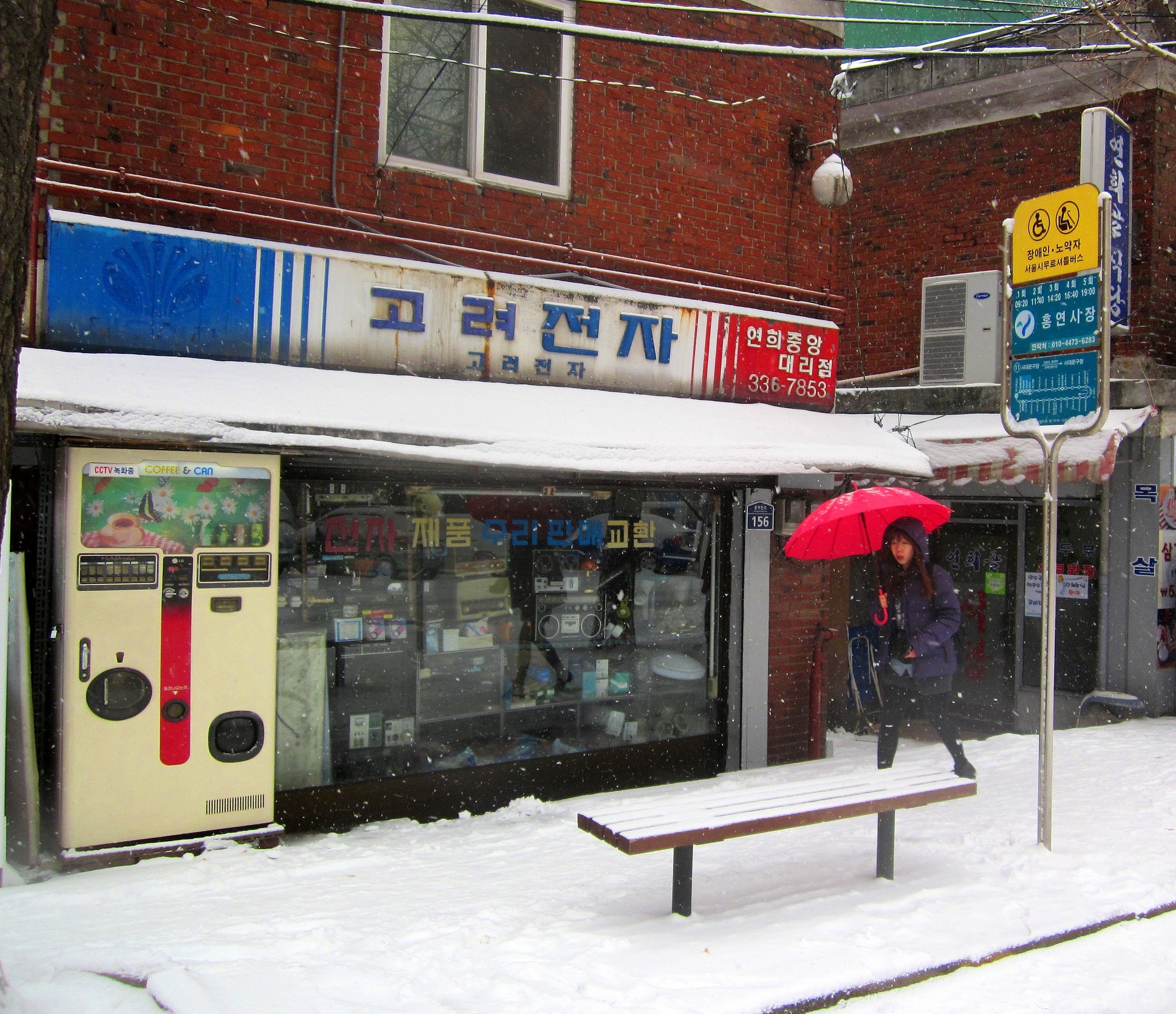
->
[363,619,385,641]
[340,713,382,750]
[439,628,459,652]
[425,617,444,655]
[385,620,407,639]
[327,618,363,642]
[580,667,596,700]
[382,716,415,747]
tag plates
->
[99,525,142,548]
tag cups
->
[108,513,143,542]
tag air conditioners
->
[919,270,1005,387]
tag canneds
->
[235,524,246,546]
[200,523,211,546]
[218,524,229,547]
[251,523,263,547]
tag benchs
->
[577,767,977,917]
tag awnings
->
[16,345,1149,483]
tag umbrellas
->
[785,479,954,625]
[467,492,578,541]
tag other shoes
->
[503,688,524,699]
[552,670,574,692]
[952,759,976,779]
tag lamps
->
[789,125,853,207]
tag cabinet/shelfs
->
[273,462,719,834]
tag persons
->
[879,515,977,782]
[503,526,574,698]
